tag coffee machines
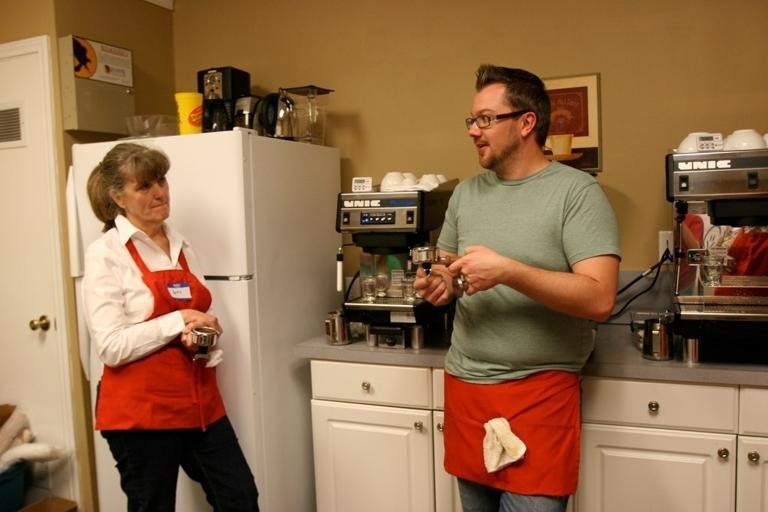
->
[334,178,459,348]
[665,148,768,364]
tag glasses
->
[466,110,530,129]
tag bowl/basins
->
[674,129,768,153]
[125,114,177,137]
[379,171,447,191]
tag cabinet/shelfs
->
[575,377,768,512]
[310,359,576,512]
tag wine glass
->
[191,326,219,361]
[701,255,724,287]
[411,245,440,274]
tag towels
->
[482,417,527,474]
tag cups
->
[401,279,417,302]
[175,92,203,135]
[544,134,574,155]
[287,89,332,147]
[374,272,388,298]
[643,318,675,361]
[325,310,353,347]
[362,275,377,301]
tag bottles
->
[234,95,262,138]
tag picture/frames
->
[542,72,602,173]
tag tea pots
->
[253,93,297,141]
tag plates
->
[544,152,583,160]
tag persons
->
[411,64,623,511]
[80,143,259,512]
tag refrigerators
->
[64,125,344,512]
